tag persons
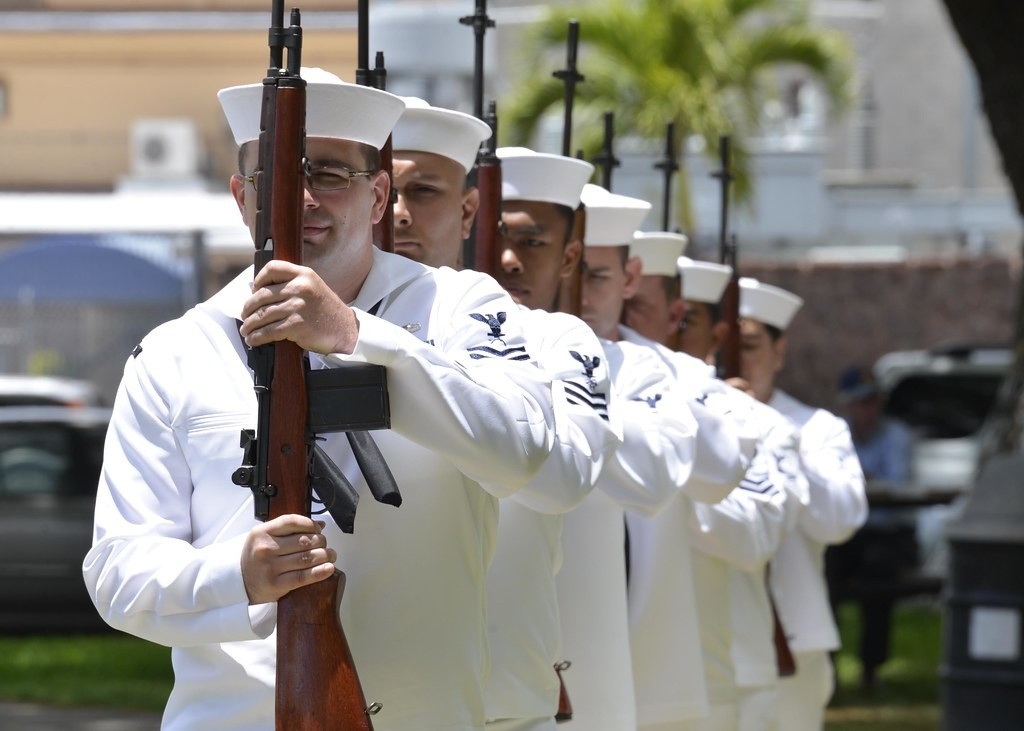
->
[581,183,758,731]
[82,65,555,731]
[823,366,916,690]
[628,230,870,731]
[373,96,624,731]
[487,145,698,731]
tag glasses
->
[241,165,376,193]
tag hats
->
[626,230,688,278]
[580,183,651,248]
[737,276,804,332]
[391,95,493,175]
[676,255,734,305]
[219,67,406,151]
[495,146,595,210]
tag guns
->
[653,122,682,238]
[550,17,588,320]
[230,1,394,731]
[711,133,745,380]
[354,1,395,252]
[457,0,503,290]
[591,110,622,190]
